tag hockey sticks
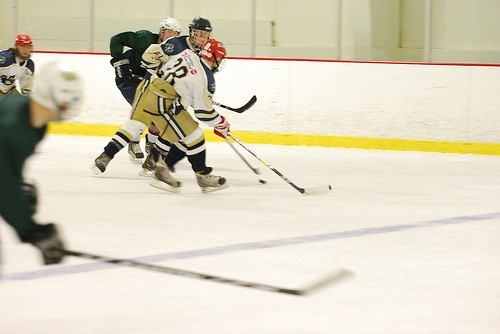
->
[226,130,334,197]
[225,136,274,185]
[57,248,354,298]
[211,94,258,114]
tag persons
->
[0,34,35,98]
[90,15,229,193]
[0,63,86,266]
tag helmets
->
[14,33,33,47]
[28,59,87,121]
[198,38,227,61]
[188,17,213,38]
[160,17,181,39]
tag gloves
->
[213,114,232,139]
[110,54,133,80]
[32,224,64,265]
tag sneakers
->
[127,140,145,164]
[94,150,111,175]
[151,158,182,192]
[194,167,229,194]
[142,151,167,176]
[145,134,155,153]
